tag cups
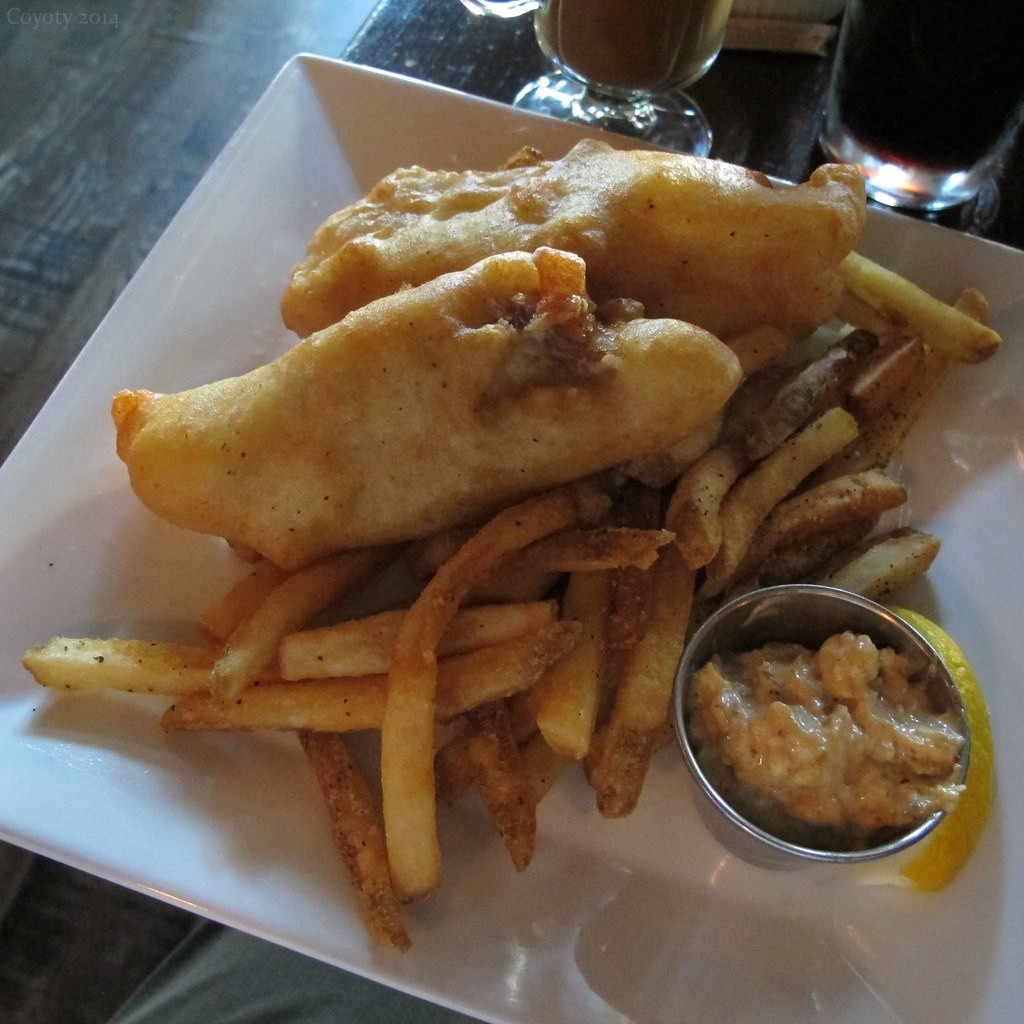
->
[813,0,1024,216]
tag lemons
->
[853,601,992,893]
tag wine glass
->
[504,0,736,178]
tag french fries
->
[22,250,1000,953]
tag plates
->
[0,54,1024,1024]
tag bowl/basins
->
[671,581,971,872]
